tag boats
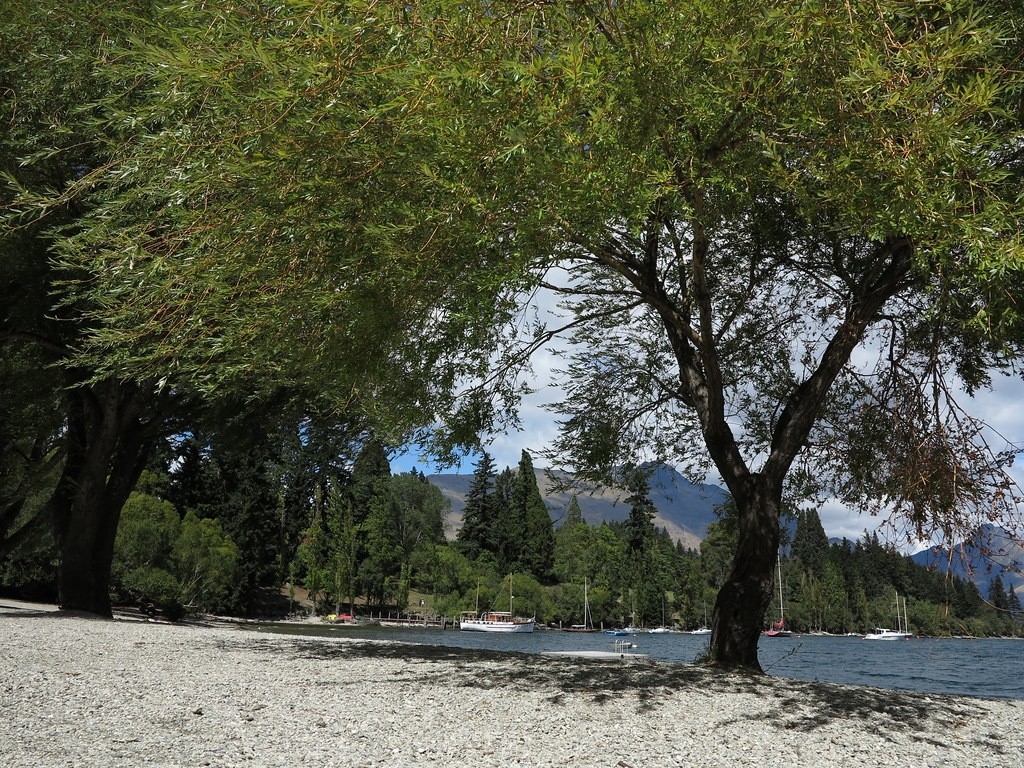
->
[691,601,712,635]
[761,617,792,637]
[648,598,669,634]
[460,573,536,633]
[625,606,640,634]
[605,628,630,636]
[563,577,598,632]
[862,628,906,641]
